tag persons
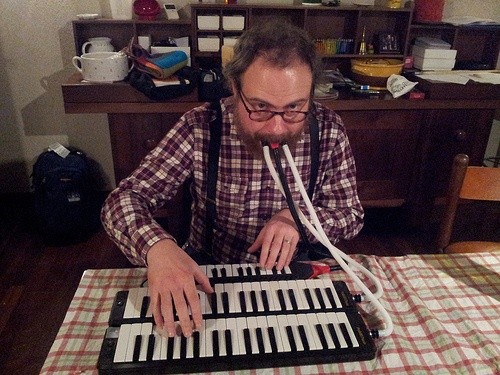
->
[100,18,364,338]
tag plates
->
[77,13,99,20]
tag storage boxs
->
[411,37,457,71]
[197,16,244,52]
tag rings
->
[284,240,291,244]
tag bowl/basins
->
[71,52,129,83]
[351,58,404,78]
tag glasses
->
[237,81,314,123]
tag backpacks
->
[29,142,106,247]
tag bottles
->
[82,37,115,55]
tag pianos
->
[97,259,377,375]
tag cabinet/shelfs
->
[61,3,500,252]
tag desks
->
[40,250,500,375]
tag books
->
[411,44,457,70]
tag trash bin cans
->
[32,145,88,244]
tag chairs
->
[434,154,500,252]
